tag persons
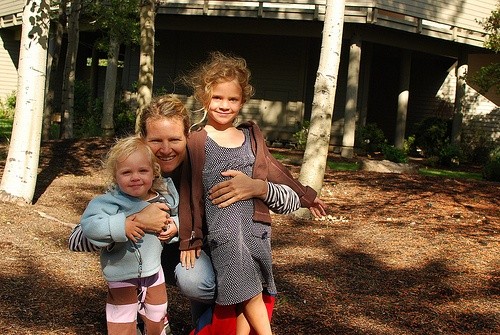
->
[69,96,301,335]
[80,137,179,335]
[179,52,327,335]
